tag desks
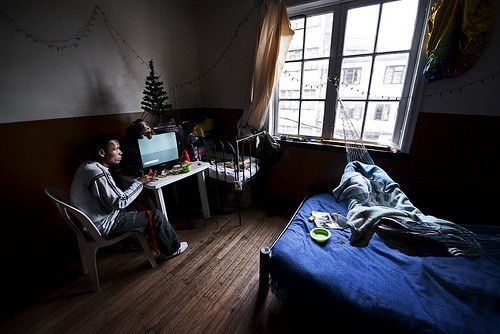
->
[126,161,212,230]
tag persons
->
[70,138,188,260]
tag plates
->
[309,227,332,243]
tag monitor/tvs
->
[137,130,180,175]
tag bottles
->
[182,150,190,163]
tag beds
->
[257,184,500,334]
[187,129,271,227]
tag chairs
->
[44,186,157,289]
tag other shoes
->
[160,242,188,260]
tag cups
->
[182,164,191,172]
